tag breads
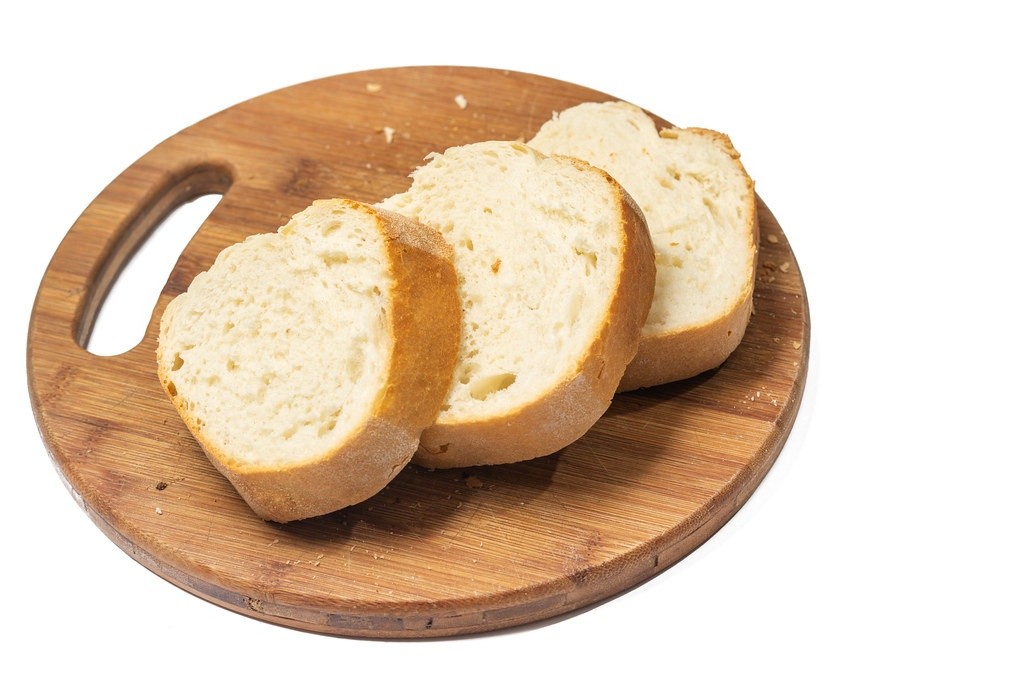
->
[156,100,759,524]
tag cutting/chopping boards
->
[26,68,810,641]
